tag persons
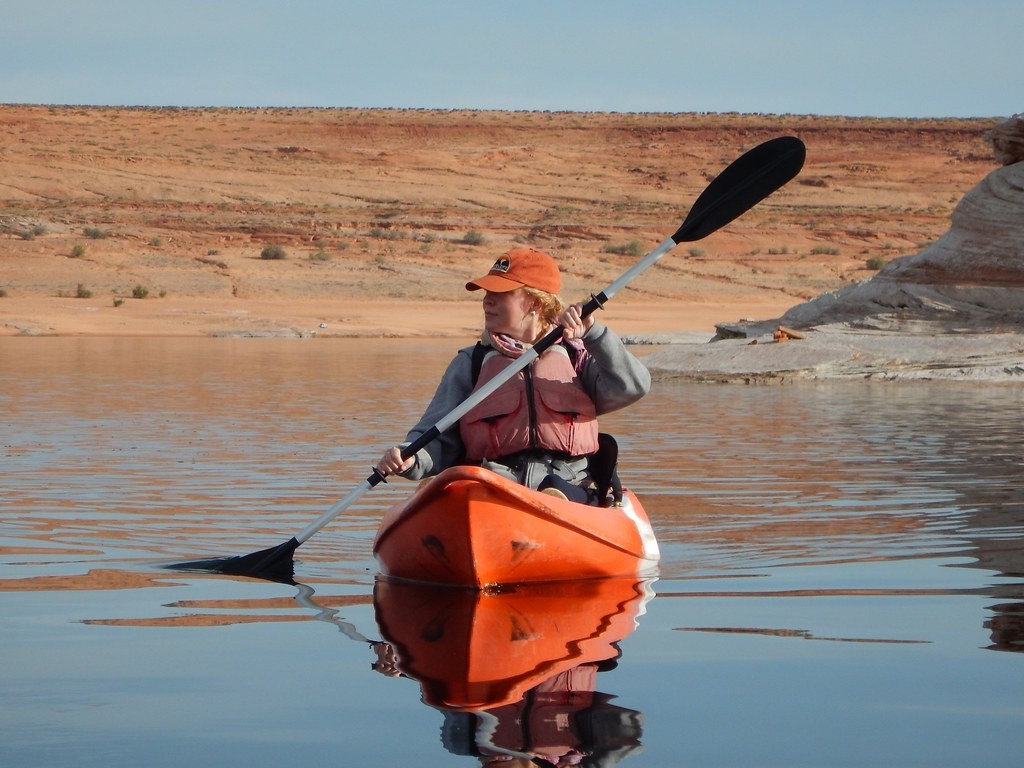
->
[371,641,647,768]
[377,249,651,503]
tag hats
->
[465,246,561,295]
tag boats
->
[373,464,663,595]
[371,570,656,712]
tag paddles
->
[220,135,806,584]
